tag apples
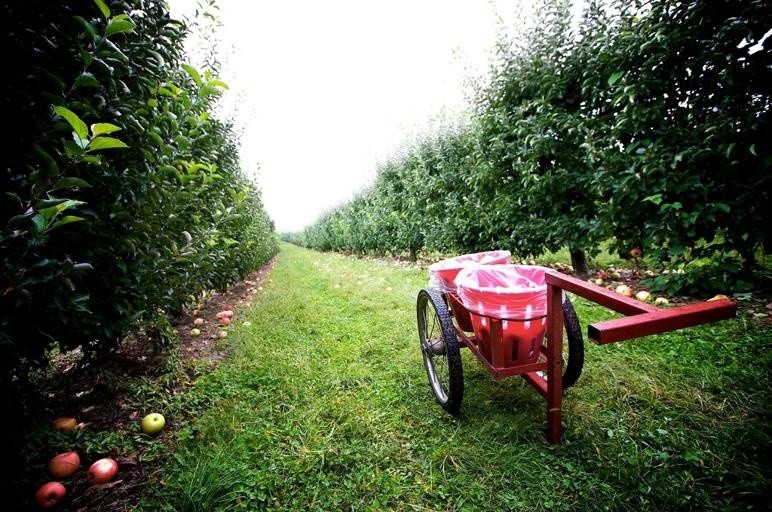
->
[140,413,164,436]
[36,481,66,510]
[189,256,276,341]
[521,260,686,307]
[47,452,81,480]
[86,456,119,485]
[53,417,77,435]
[631,247,644,257]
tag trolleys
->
[414,246,748,444]
